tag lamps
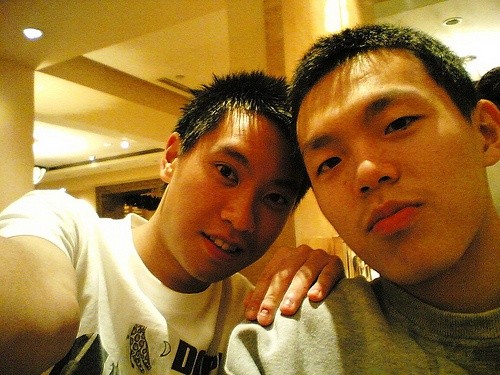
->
[32,165,47,185]
[20,27,44,42]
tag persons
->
[472,68,500,219]
[217,21,500,373]
[0,66,346,375]
